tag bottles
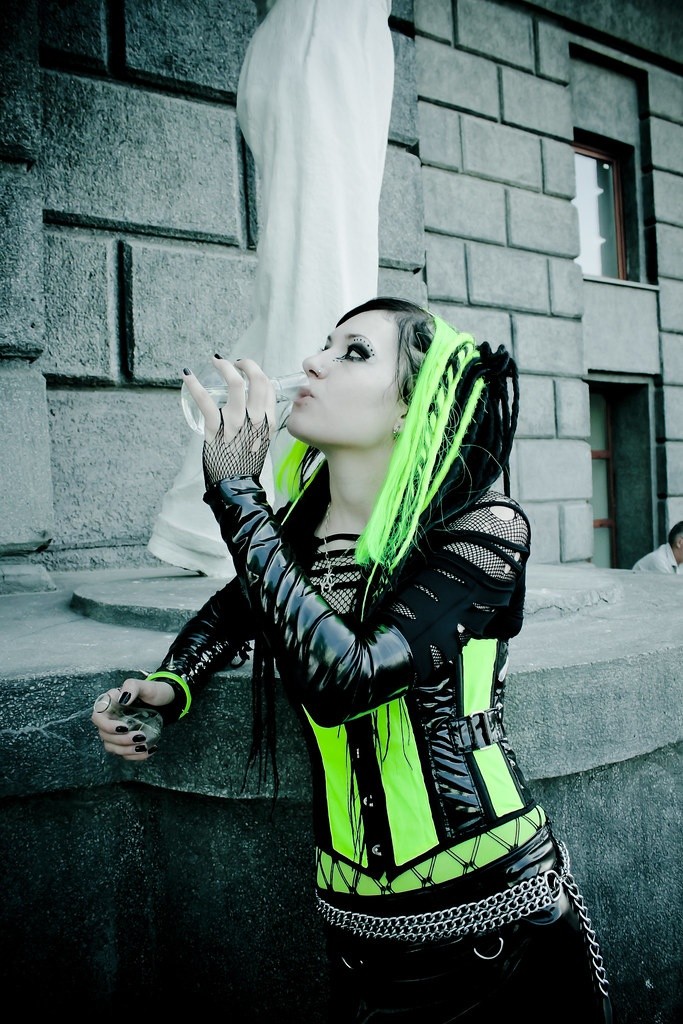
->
[182,371,311,433]
[94,693,164,749]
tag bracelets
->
[145,672,191,719]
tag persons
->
[93,296,610,1024]
[633,521,683,574]
[147,0,395,572]
